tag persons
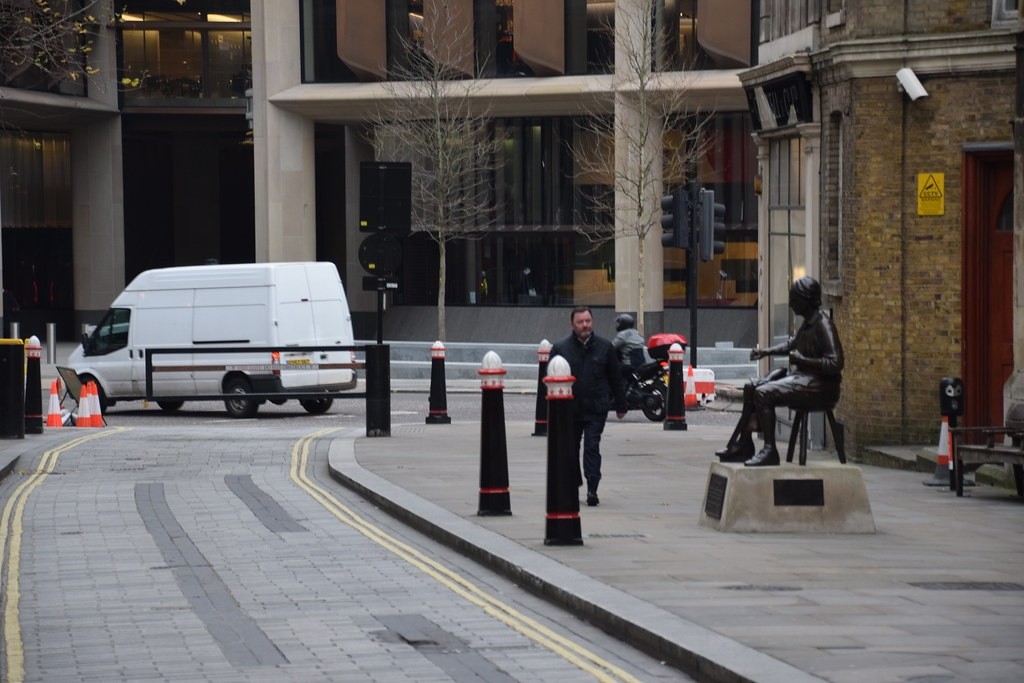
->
[612,314,646,367]
[548,308,628,506]
[716,277,845,466]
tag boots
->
[587,480,599,506]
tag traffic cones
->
[55,376,64,409]
[85,380,107,429]
[923,414,975,487]
[73,384,93,428]
[46,379,65,428]
[683,364,705,412]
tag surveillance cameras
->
[896,67,928,101]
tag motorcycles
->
[624,333,689,421]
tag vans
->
[55,260,358,420]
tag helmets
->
[616,314,634,332]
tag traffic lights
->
[660,183,691,250]
[700,189,728,263]
